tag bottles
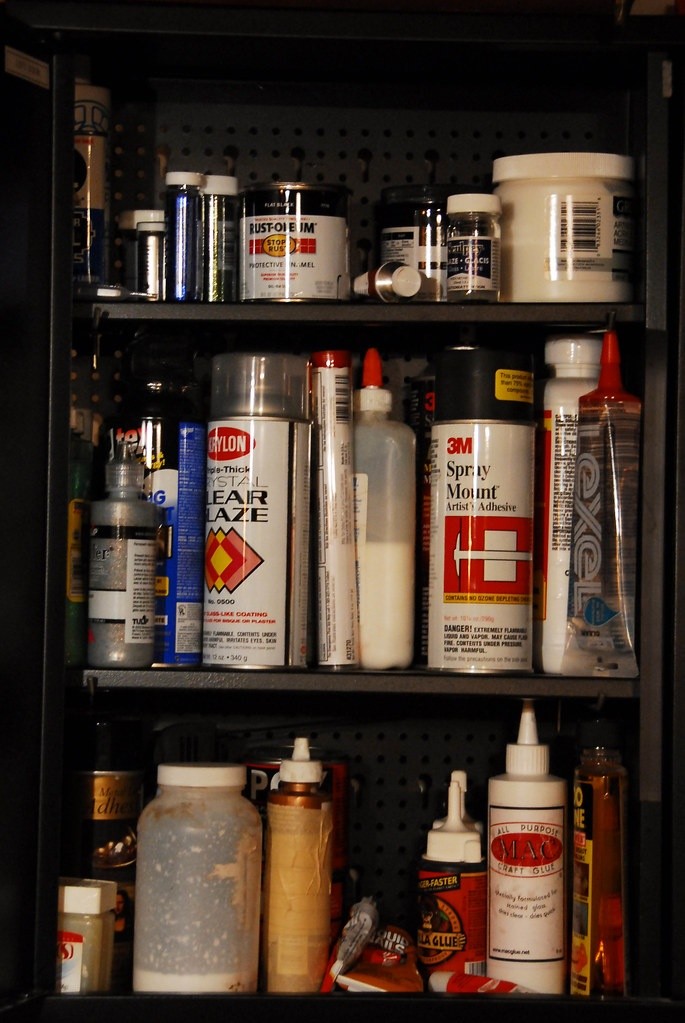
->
[70,689,142,993]
[264,736,333,993]
[567,330,640,676]
[198,175,239,302]
[416,770,487,996]
[488,698,567,996]
[132,762,262,993]
[56,878,116,992]
[86,441,157,668]
[404,352,437,668]
[430,347,534,673]
[569,742,625,999]
[350,349,417,670]
[163,172,204,302]
[445,194,502,301]
[116,210,165,293]
[532,338,599,673]
[113,379,205,667]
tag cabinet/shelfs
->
[27,1,685,1023]
